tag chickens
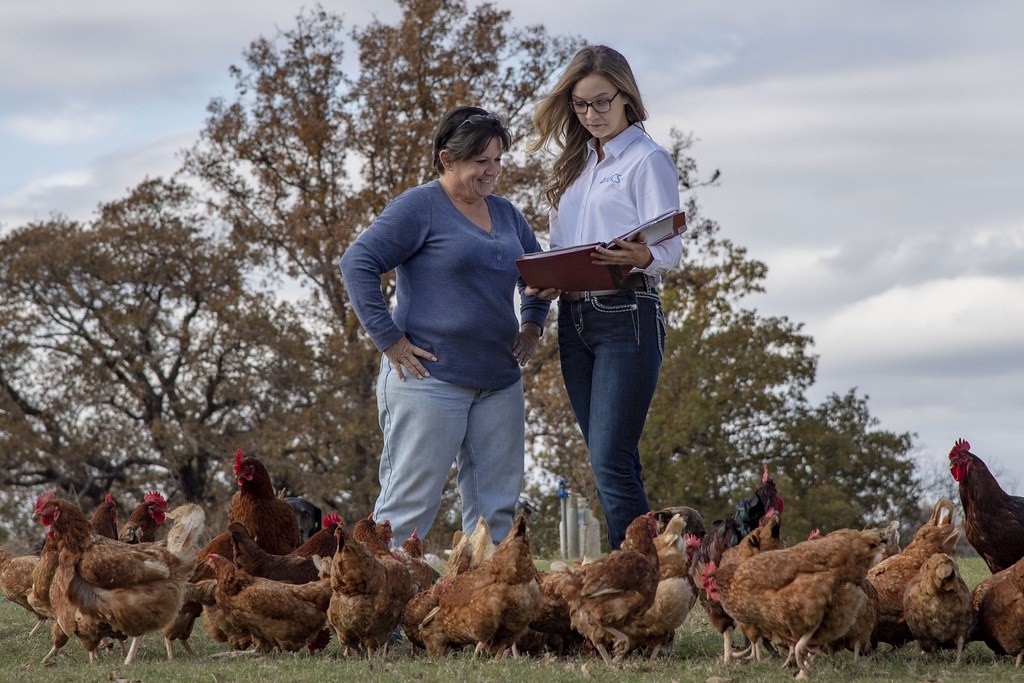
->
[1,438,1024,679]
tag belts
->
[558,273,656,306]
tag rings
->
[638,237,647,244]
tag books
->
[513,208,690,293]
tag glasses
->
[449,112,501,135]
[567,89,622,115]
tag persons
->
[523,43,683,558]
[339,102,549,566]
